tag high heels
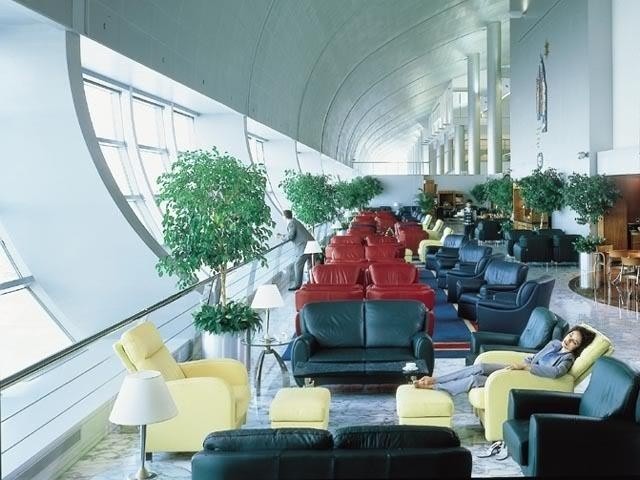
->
[478,441,508,460]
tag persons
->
[413,326,596,396]
[277,210,315,290]
[461,199,475,241]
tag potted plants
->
[563,172,623,290]
[153,148,263,369]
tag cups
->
[406,362,417,370]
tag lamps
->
[109,369,178,480]
[251,284,284,342]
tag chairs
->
[286,206,586,334]
[466,305,570,365]
[113,320,250,460]
[469,323,614,440]
[503,356,639,478]
[593,244,640,319]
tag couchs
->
[289,299,436,387]
[191,445,472,480]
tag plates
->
[402,366,419,371]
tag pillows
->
[335,426,461,448]
[200,425,335,451]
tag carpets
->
[568,268,640,313]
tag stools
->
[271,387,330,431]
[395,384,453,426]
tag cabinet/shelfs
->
[513,183,549,231]
[438,190,469,209]
